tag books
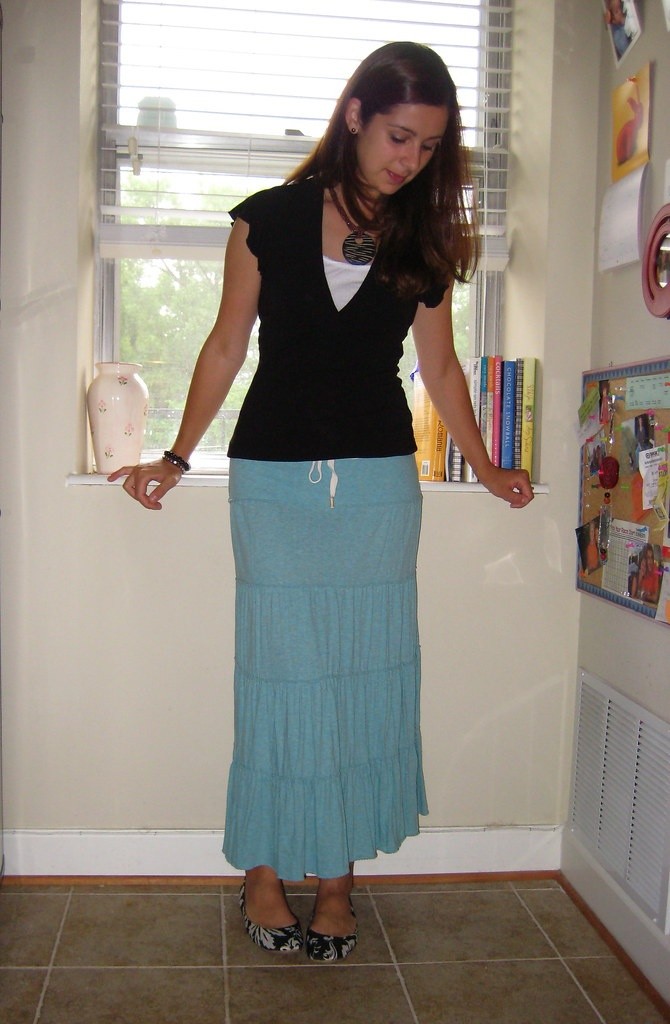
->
[408,351,537,484]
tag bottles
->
[87,362,149,474]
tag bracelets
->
[162,450,191,475]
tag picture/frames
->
[602,0,643,70]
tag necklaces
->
[328,184,386,267]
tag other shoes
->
[238,879,304,951]
[305,896,359,962]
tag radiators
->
[570,666,670,936]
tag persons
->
[108,41,536,962]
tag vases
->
[86,361,149,475]
